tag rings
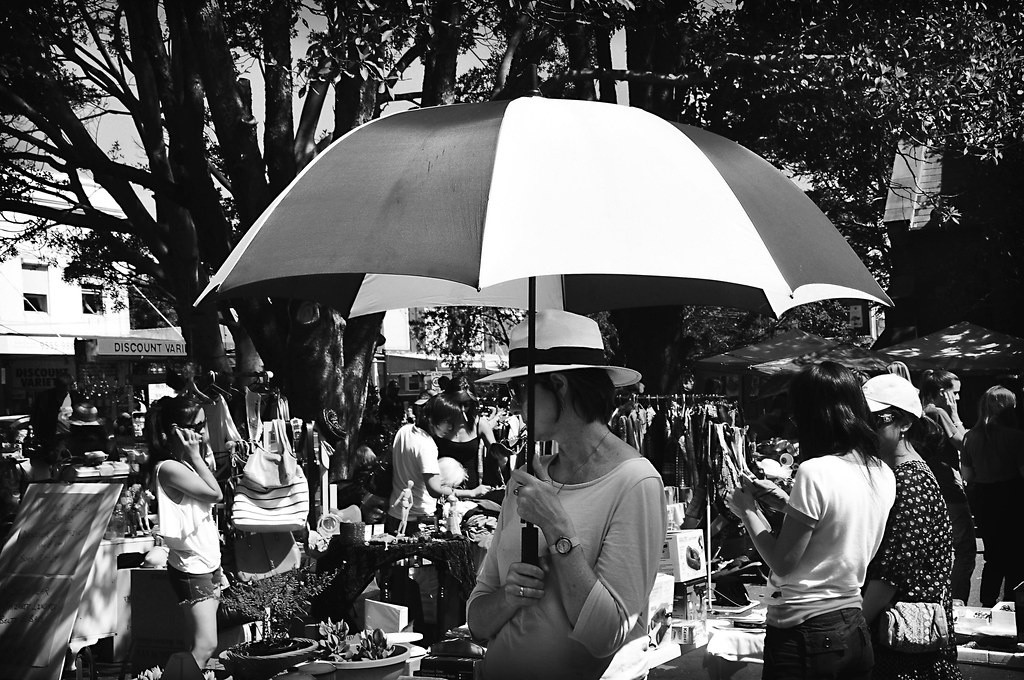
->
[513,485,523,496]
[520,587,524,597]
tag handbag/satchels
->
[233,421,310,583]
[353,453,395,525]
[881,602,949,652]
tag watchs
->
[548,535,580,555]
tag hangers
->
[614,393,722,404]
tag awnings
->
[85,334,187,356]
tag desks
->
[314,536,482,649]
[706,609,1024,680]
[69,530,168,680]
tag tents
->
[693,326,938,396]
[876,321,1024,374]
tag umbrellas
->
[191,61,895,565]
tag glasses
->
[789,414,798,428]
[179,417,207,433]
[876,413,898,426]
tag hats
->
[861,374,922,419]
[474,308,642,388]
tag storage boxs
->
[989,601,1015,625]
[957,606,992,624]
[659,528,707,582]
[647,572,709,669]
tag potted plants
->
[292,617,410,680]
[192,559,347,680]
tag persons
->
[142,396,224,667]
[957,385,1023,610]
[724,361,896,679]
[861,374,963,680]
[391,480,414,535]
[908,369,977,606]
[384,375,530,539]
[464,310,667,680]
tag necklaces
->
[551,431,610,494]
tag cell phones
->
[171,425,189,441]
[741,476,761,504]
